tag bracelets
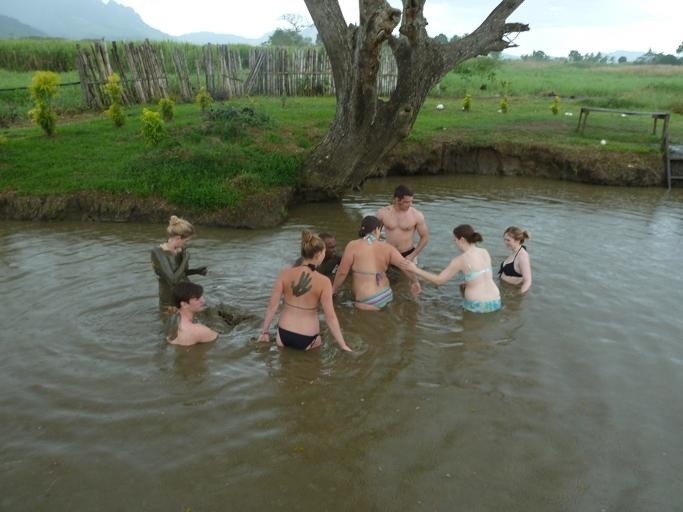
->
[262,331,269,334]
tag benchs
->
[576,107,670,139]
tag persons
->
[150,215,208,309]
[375,184,428,265]
[332,215,422,311]
[164,281,218,346]
[292,232,341,286]
[497,226,532,293]
[400,224,502,313]
[254,230,353,352]
